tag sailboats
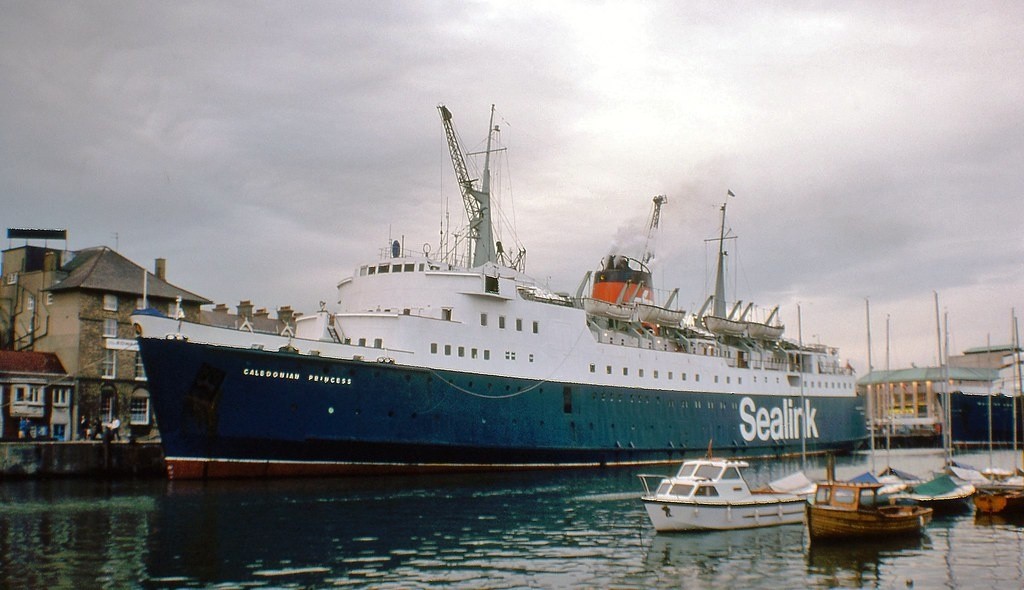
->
[761,293,1024,514]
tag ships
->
[130,105,875,486]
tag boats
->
[581,296,634,321]
[634,458,824,532]
[638,303,686,328]
[749,322,784,342]
[806,483,934,545]
[704,316,749,335]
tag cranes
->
[589,194,666,334]
[436,103,530,272]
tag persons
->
[80,416,122,443]
[19,417,32,441]
